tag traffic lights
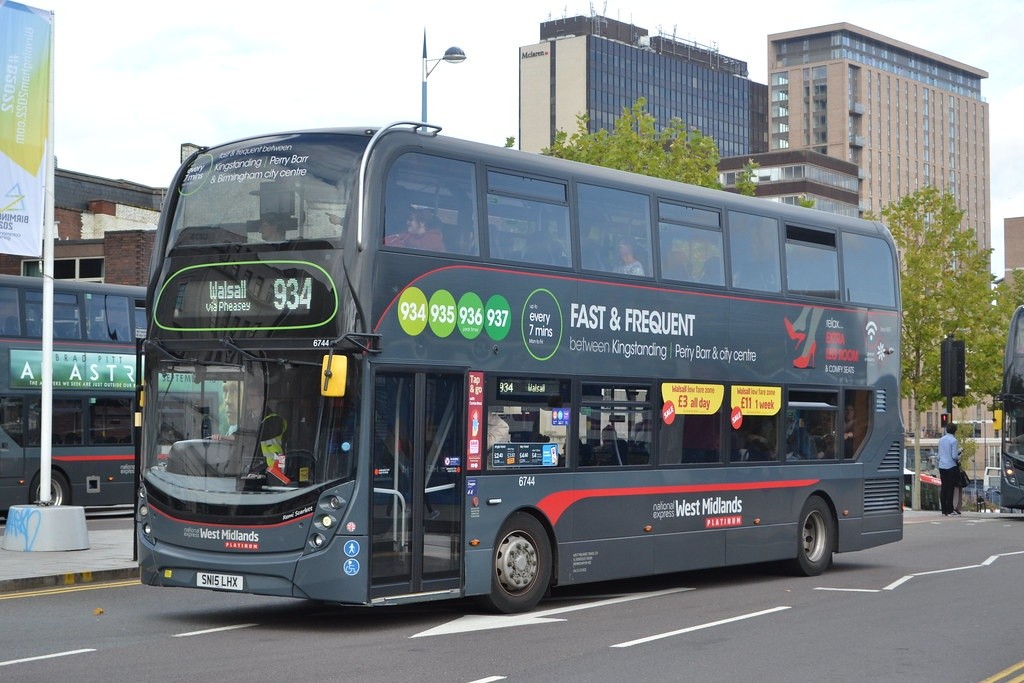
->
[940,414,951,427]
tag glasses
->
[247,394,263,398]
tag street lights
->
[419,27,467,134]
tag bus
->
[134,121,904,614]
[991,306,1024,510]
[0,274,149,516]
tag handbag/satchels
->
[954,467,970,487]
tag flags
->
[0,0,51,257]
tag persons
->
[211,382,287,467]
[259,211,286,242]
[936,423,961,516]
[325,165,410,227]
[487,408,509,470]
[1011,433,1024,443]
[612,236,645,276]
[384,209,445,251]
[222,381,243,436]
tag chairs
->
[4,315,132,344]
[450,205,724,287]
[563,428,857,467]
[11,432,132,446]
